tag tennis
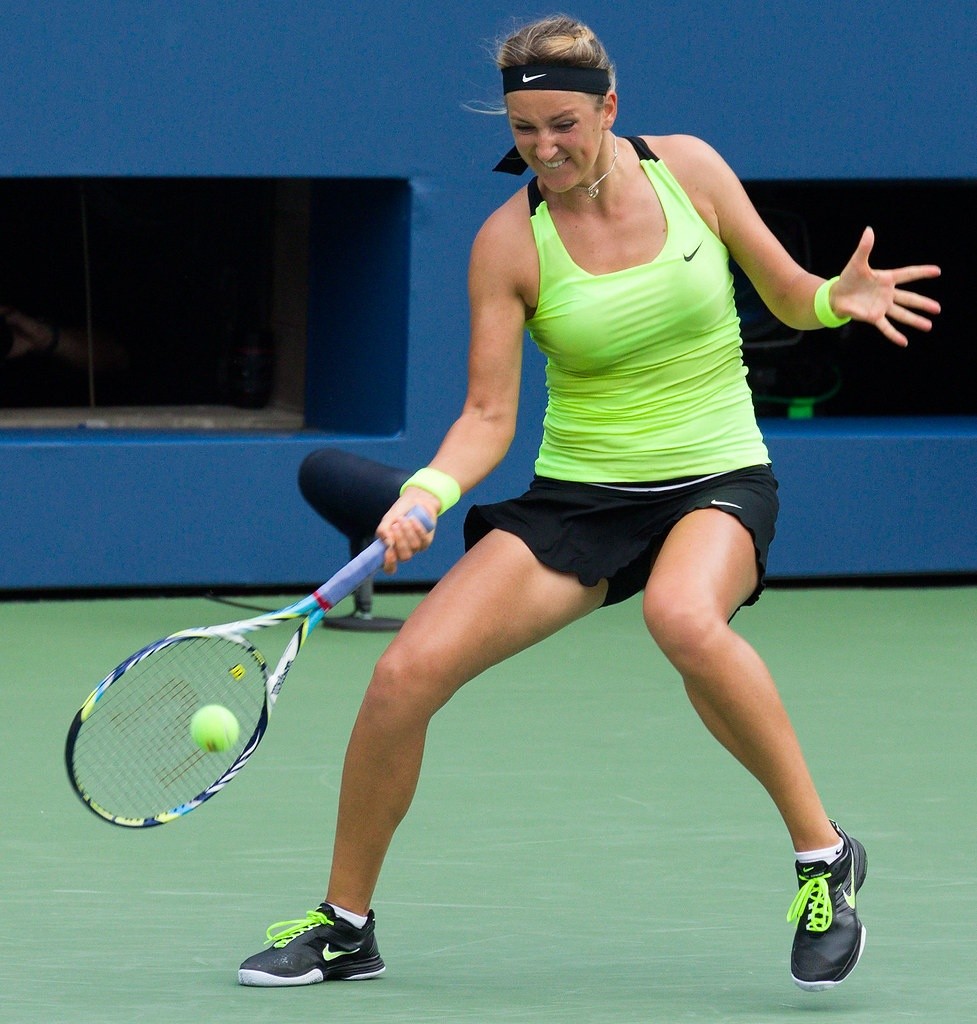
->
[189,704,241,753]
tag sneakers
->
[787,819,867,992]
[238,903,385,988]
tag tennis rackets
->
[60,504,439,832]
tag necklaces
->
[572,134,618,203]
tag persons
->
[236,15,941,993]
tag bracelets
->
[815,277,852,329]
[400,467,461,519]
